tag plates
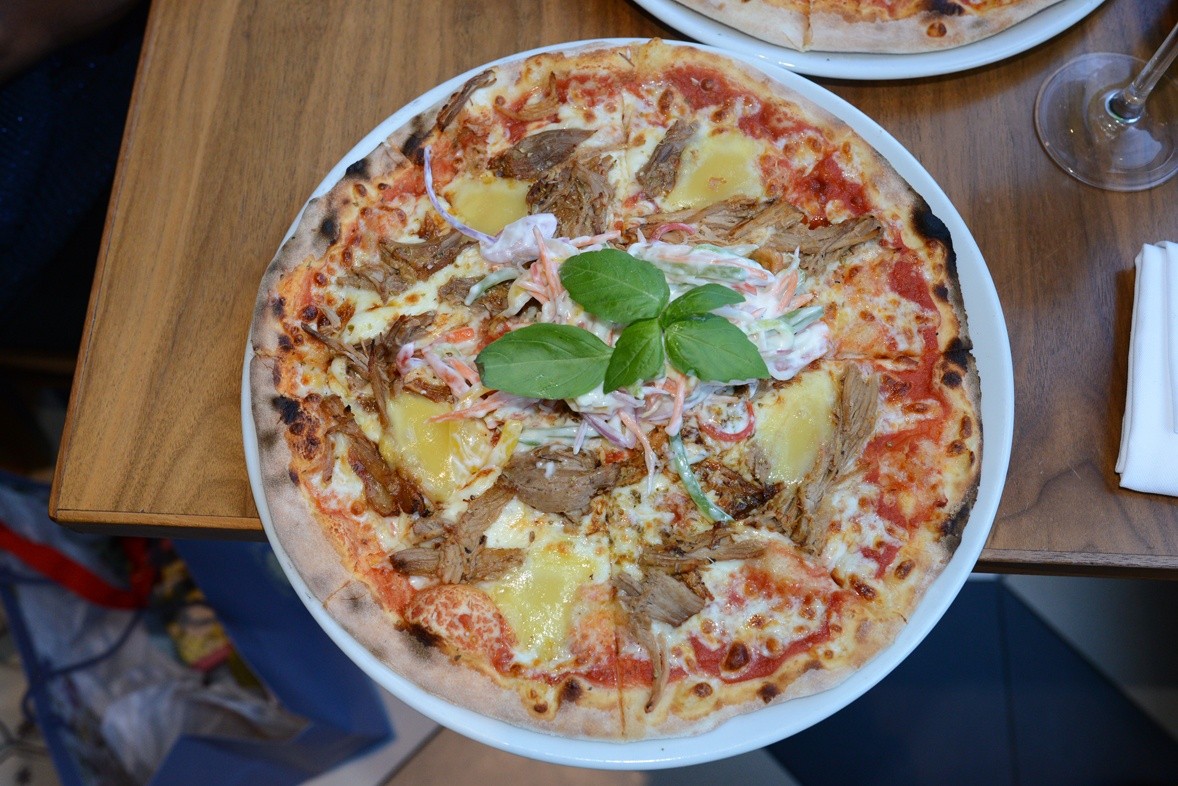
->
[241,37,1016,772]
[634,0,1106,80]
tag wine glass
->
[1034,24,1178,191]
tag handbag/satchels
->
[1,483,397,786]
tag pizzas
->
[675,0,1063,54]
[243,37,980,745]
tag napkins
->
[1114,240,1178,496]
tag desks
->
[46,0,1178,580]
